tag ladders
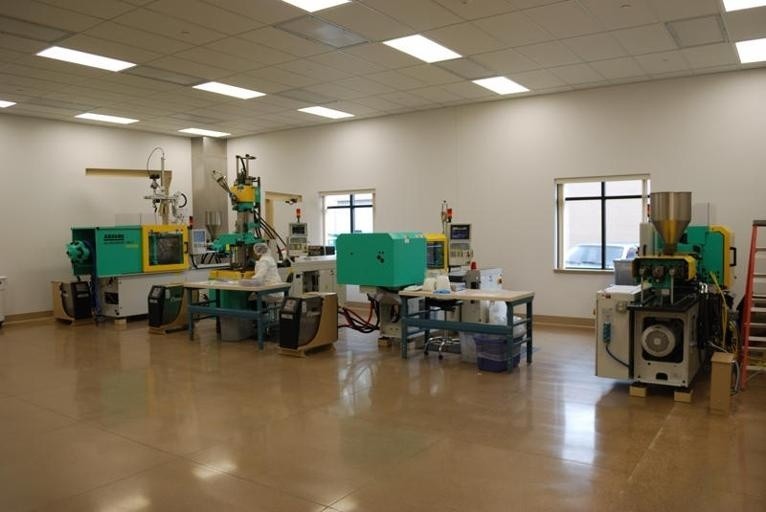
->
[738,220,766,389]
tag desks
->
[183,278,292,350]
[398,286,535,375]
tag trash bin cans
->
[473,332,526,372]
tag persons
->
[248,243,285,328]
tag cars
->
[564,242,640,270]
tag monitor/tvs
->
[290,223,307,237]
[449,223,472,242]
[189,229,206,244]
